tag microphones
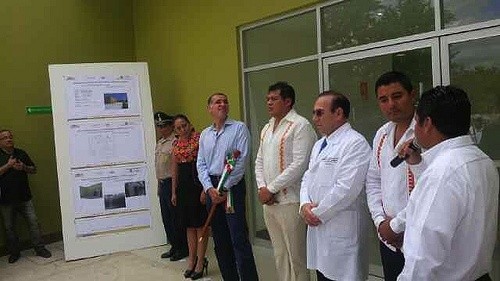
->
[390,137,420,168]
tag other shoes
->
[38,249,51,257]
[170,252,189,261]
[161,247,175,258]
[9,252,21,264]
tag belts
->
[209,176,221,181]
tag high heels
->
[190,258,209,280]
[184,256,198,278]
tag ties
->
[319,139,327,153]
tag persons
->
[172,113,210,280]
[0,128,52,264]
[196,92,261,281]
[251,81,321,281]
[396,84,500,281]
[298,86,371,281]
[154,110,190,262]
[363,69,433,281]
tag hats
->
[155,112,176,127]
